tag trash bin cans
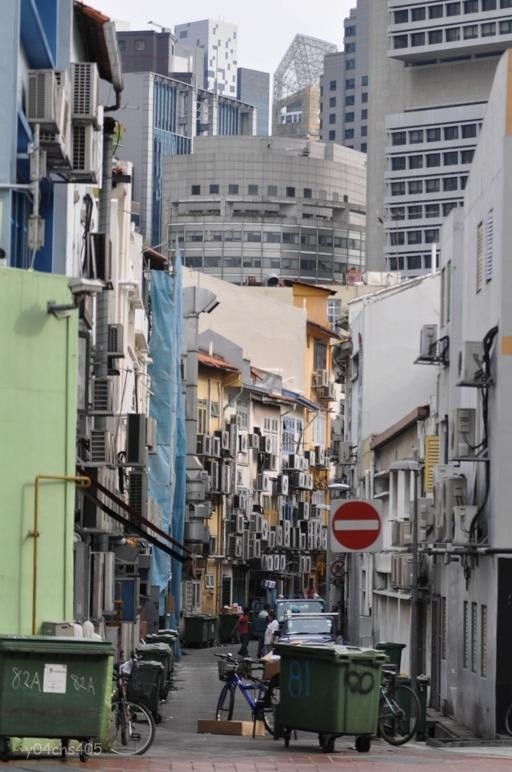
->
[221,614,244,643]
[0,634,115,761]
[277,640,428,751]
[125,629,178,724]
[184,614,217,648]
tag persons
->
[261,611,280,659]
[256,603,272,657]
[230,606,251,657]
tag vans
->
[279,616,341,650]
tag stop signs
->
[329,499,384,552]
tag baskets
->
[219,661,251,681]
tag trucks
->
[254,598,327,638]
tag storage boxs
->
[197,719,265,736]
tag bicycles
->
[214,652,285,737]
[375,669,422,746]
[108,663,156,759]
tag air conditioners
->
[22,59,328,574]
[389,323,490,591]
